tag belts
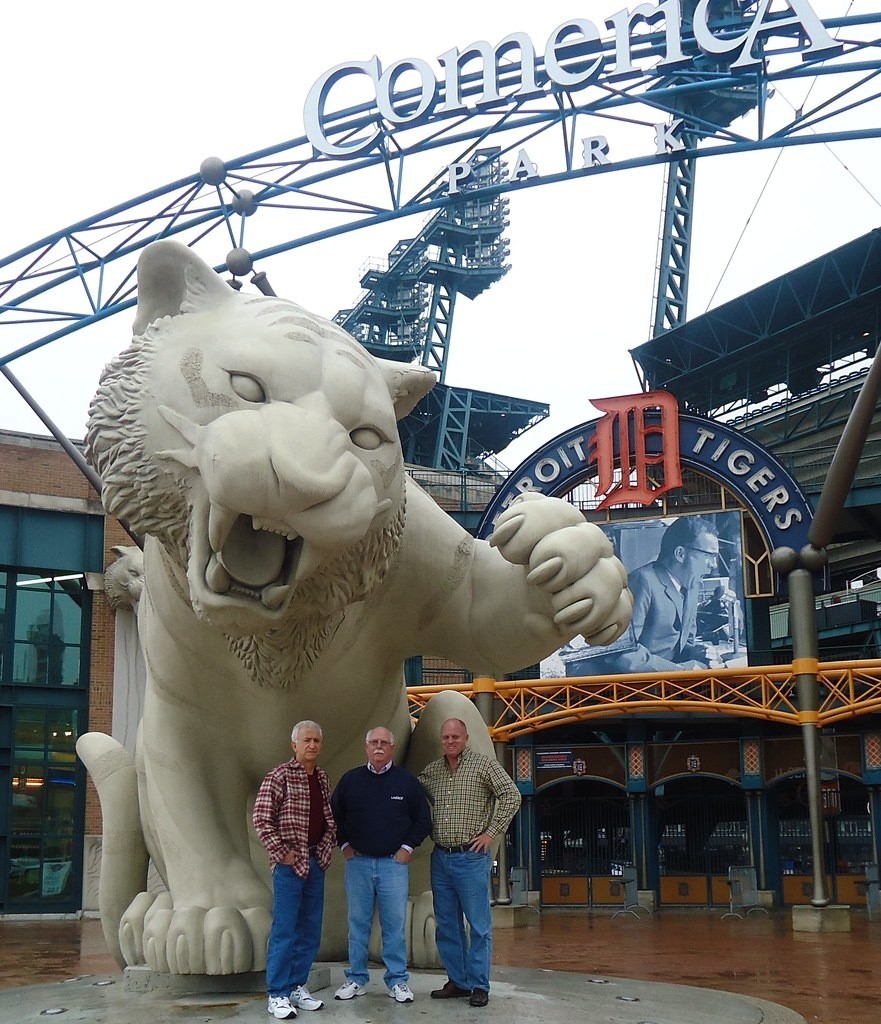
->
[435,843,472,853]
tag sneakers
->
[334,981,366,1000]
[388,984,414,1003]
[289,984,324,1011]
[267,995,297,1019]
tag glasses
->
[682,545,720,562]
[367,739,389,745]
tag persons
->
[417,718,522,1007]
[329,726,435,1002]
[607,516,719,674]
[253,721,338,1019]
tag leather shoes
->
[431,981,471,998]
[470,988,488,1006]
